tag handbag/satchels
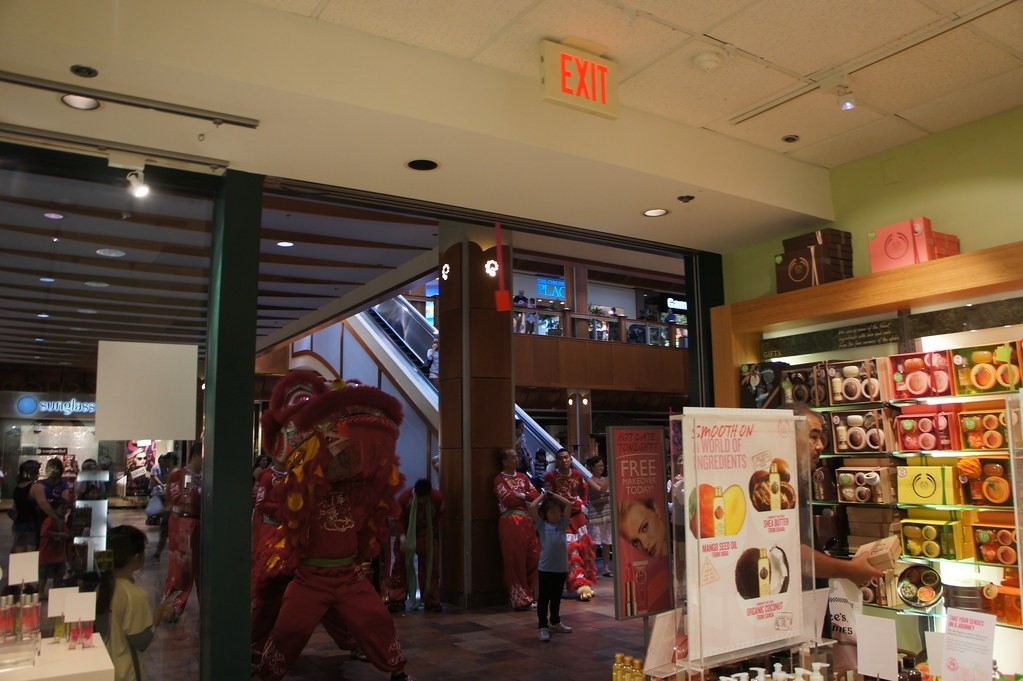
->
[828,578,863,671]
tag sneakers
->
[549,622,571,633]
[537,627,549,641]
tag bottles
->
[620,655,634,681]
[612,652,624,681]
[630,658,647,681]
[0,593,94,651]
[769,463,781,511]
[713,485,725,537]
[758,547,770,598]
[863,656,921,681]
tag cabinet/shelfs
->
[807,400,898,618]
[890,388,1023,630]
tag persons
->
[148,442,202,629]
[427,338,439,390]
[513,289,528,333]
[92,524,160,681]
[606,307,618,340]
[529,488,572,642]
[493,447,539,611]
[667,453,686,577]
[617,497,667,559]
[73,459,106,500]
[97,455,114,500]
[586,455,614,578]
[525,298,536,334]
[544,448,589,505]
[664,309,677,340]
[4,459,70,603]
[515,419,548,494]
[249,454,446,681]
[779,401,885,588]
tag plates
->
[739,344,1021,608]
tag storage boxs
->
[867,217,958,274]
[774,227,853,294]
[741,341,1023,607]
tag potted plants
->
[589,305,603,339]
[542,299,563,336]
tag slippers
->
[602,571,615,577]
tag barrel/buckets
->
[941,581,994,616]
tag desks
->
[0,633,115,681]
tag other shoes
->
[351,648,366,661]
[520,602,537,610]
[391,672,413,681]
[388,602,405,612]
[425,603,442,613]
[165,616,177,623]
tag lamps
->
[837,85,856,110]
[127,170,149,197]
